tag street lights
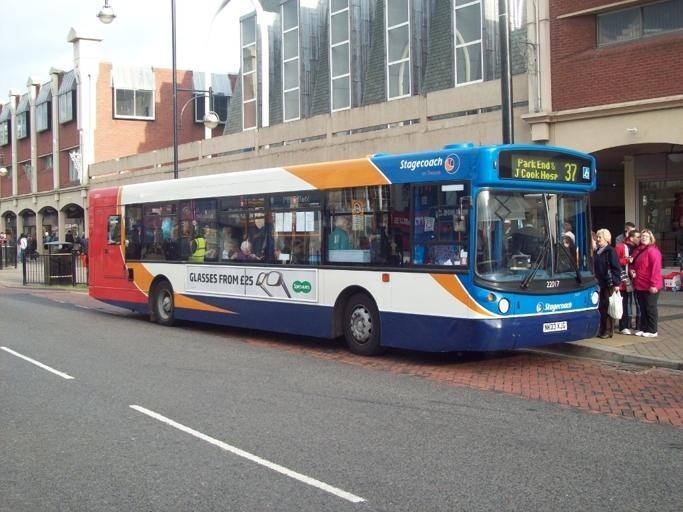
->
[0,151,7,178]
[95,1,218,179]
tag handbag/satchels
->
[619,270,633,293]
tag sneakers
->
[619,328,631,335]
[635,331,643,336]
[643,332,657,338]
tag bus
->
[85,141,600,362]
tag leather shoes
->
[599,334,612,339]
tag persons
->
[510,211,545,263]
[128,213,283,264]
[560,221,664,339]
[424,216,461,264]
[369,216,403,263]
[0,229,87,271]
[329,213,352,249]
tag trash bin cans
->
[2,246,16,266]
[44,241,73,286]
